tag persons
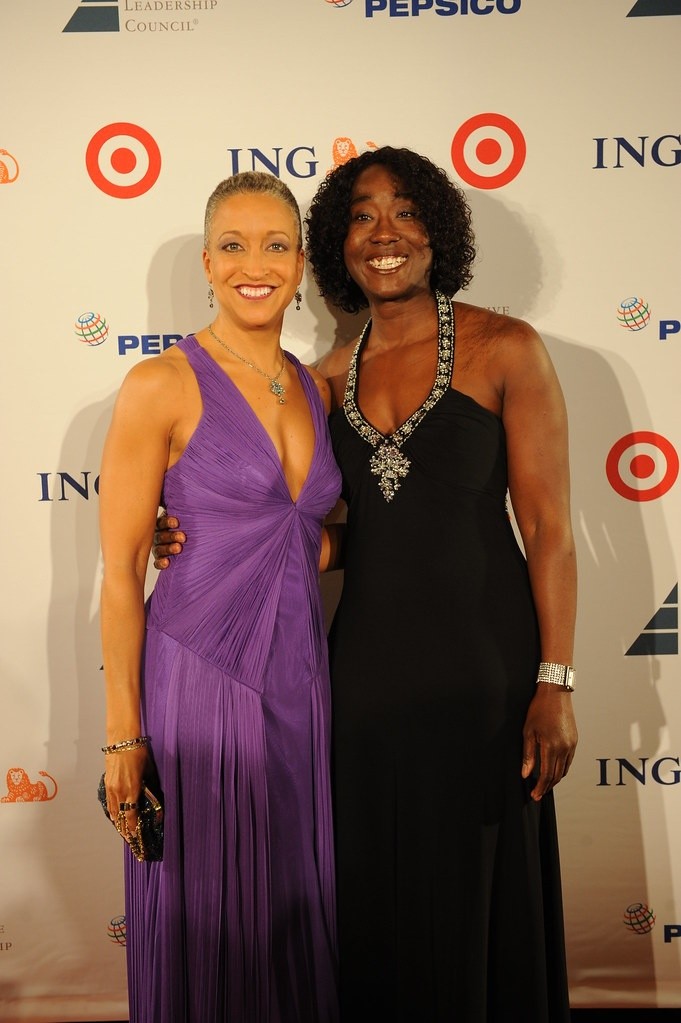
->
[150,145,579,1023]
[89,171,341,1023]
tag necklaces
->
[208,325,287,404]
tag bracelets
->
[101,736,151,754]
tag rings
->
[124,803,136,810]
[118,803,125,811]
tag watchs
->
[537,662,576,692]
